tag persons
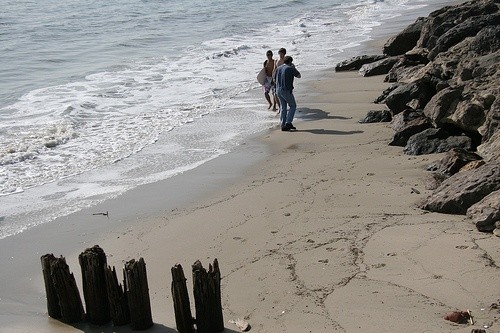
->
[274,48,290,114]
[263,50,276,110]
[273,56,301,130]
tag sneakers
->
[285,123,296,129]
[281,126,290,131]
